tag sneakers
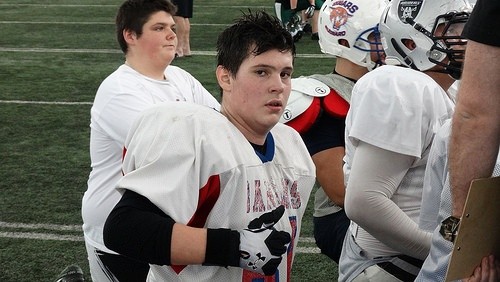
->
[54,265,85,282]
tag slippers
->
[174,53,192,59]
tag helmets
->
[318,0,390,72]
[379,0,477,71]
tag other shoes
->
[293,29,302,42]
[311,32,320,41]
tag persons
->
[337,0,500,282]
[53,0,221,282]
[102,7,316,282]
[274,0,389,265]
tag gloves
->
[305,6,315,19]
[204,205,291,277]
